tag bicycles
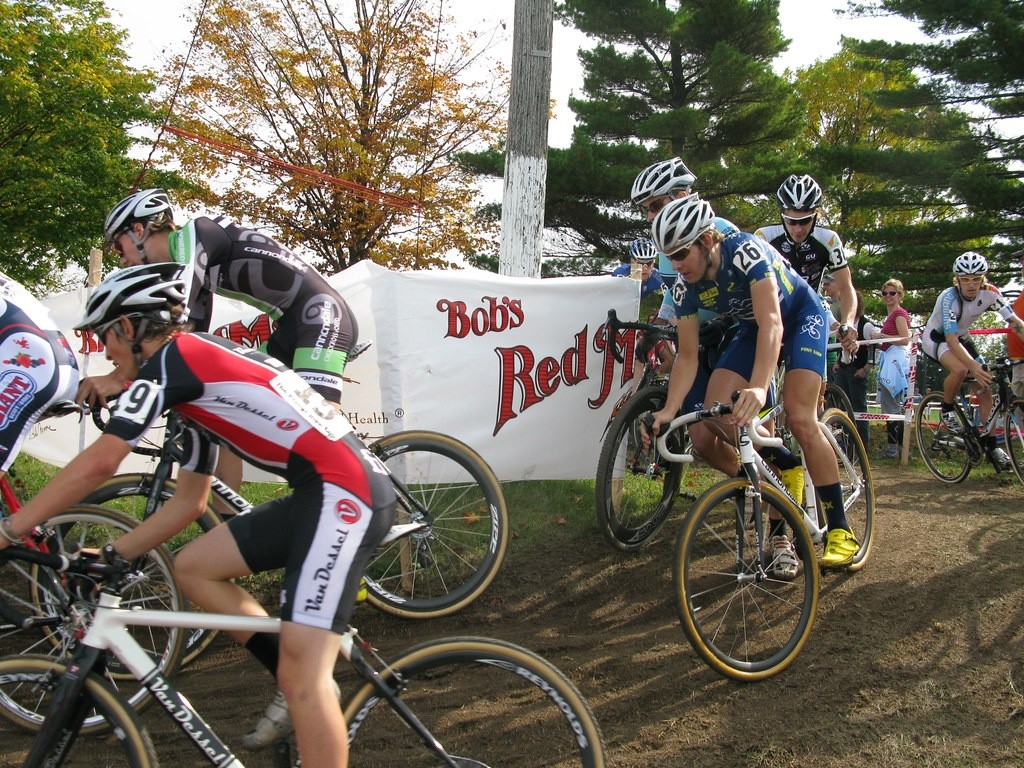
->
[27,339,510,682]
[822,324,857,466]
[0,521,610,768]
[915,357,1024,485]
[641,390,877,682]
[0,400,186,734]
[594,308,734,554]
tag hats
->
[821,273,835,283]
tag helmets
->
[631,156,698,204]
[631,238,658,263]
[953,251,988,275]
[71,262,187,337]
[652,192,715,254]
[776,174,822,211]
[104,189,170,242]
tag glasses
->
[641,192,677,212]
[636,261,655,266]
[94,312,144,345]
[113,227,133,253]
[664,225,713,260]
[882,291,898,296]
[959,276,983,283]
[781,212,817,225]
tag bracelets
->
[864,367,868,375]
[881,333,885,339]
[0,517,27,544]
[102,544,117,566]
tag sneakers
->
[941,409,961,432]
[880,449,917,460]
[821,527,860,568]
[243,676,342,749]
[992,448,1012,468]
[782,454,803,508]
[769,535,798,580]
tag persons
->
[612,157,861,579]
[833,251,1024,471]
[0,262,397,768]
[0,270,80,481]
[75,187,367,604]
